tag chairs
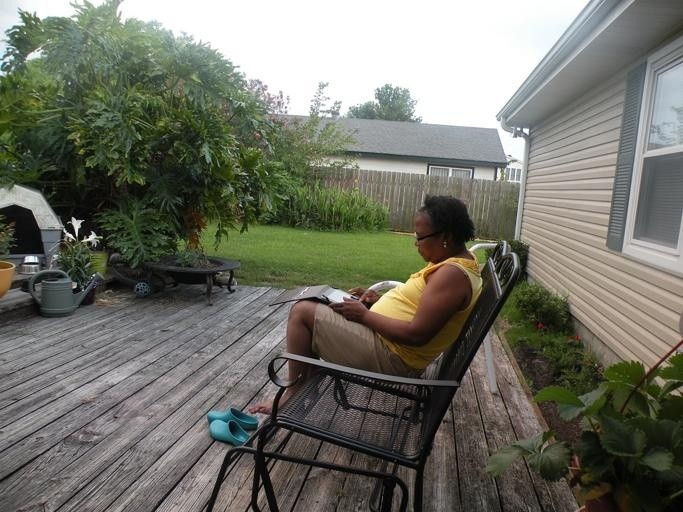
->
[206,239,521,511]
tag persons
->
[247,193,485,415]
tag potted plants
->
[0,214,17,299]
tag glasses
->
[415,230,438,241]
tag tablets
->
[322,288,360,306]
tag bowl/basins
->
[17,255,41,275]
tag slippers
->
[207,407,258,429]
[210,420,253,447]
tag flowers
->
[47,216,103,287]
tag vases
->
[71,287,96,305]
